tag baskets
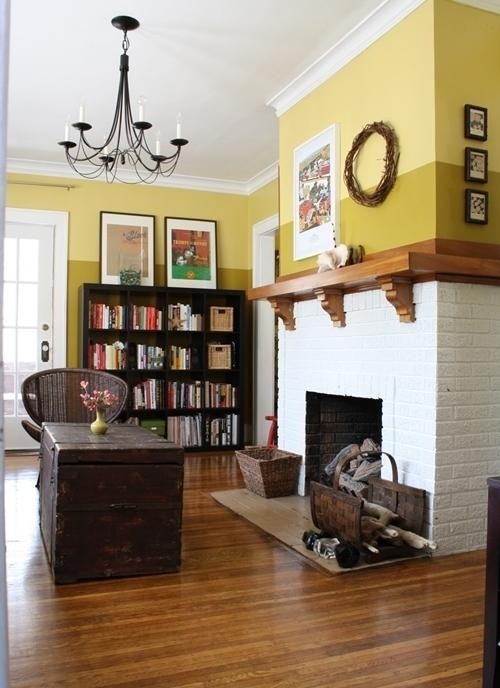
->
[234,447,301,498]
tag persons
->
[171,229,208,267]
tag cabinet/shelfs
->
[167,287,256,452]
[77,281,168,439]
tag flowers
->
[78,380,117,411]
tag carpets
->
[201,487,434,577]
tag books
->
[85,298,240,447]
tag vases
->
[91,407,107,432]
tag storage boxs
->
[36,420,185,587]
[141,421,165,436]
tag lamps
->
[58,16,188,188]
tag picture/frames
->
[463,103,488,141]
[164,216,219,290]
[98,210,156,288]
[292,125,340,264]
[465,190,488,224]
[464,148,487,183]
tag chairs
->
[19,369,131,488]
